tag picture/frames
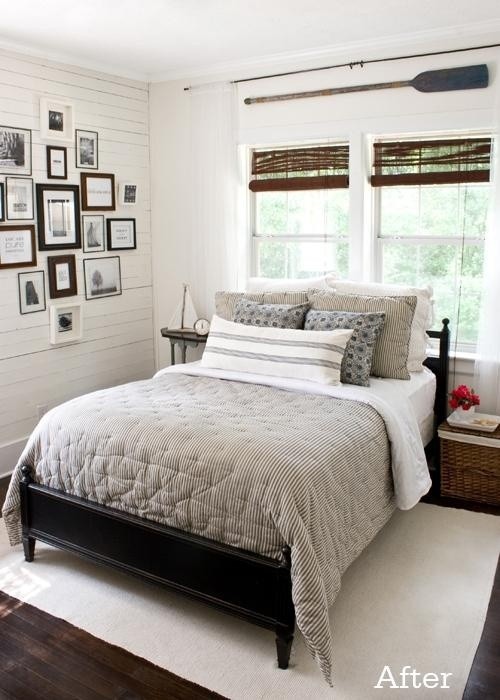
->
[1,97,136,342]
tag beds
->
[1,281,450,687]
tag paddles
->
[244,64,489,104]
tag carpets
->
[0,503,499,698]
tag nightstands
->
[162,329,207,365]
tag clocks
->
[194,319,210,335]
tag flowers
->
[447,376,482,418]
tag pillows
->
[326,277,432,370]
[305,291,416,380]
[306,307,386,385]
[232,299,311,351]
[247,273,334,291]
[215,291,305,347]
[202,314,353,384]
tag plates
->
[447,411,500,432]
